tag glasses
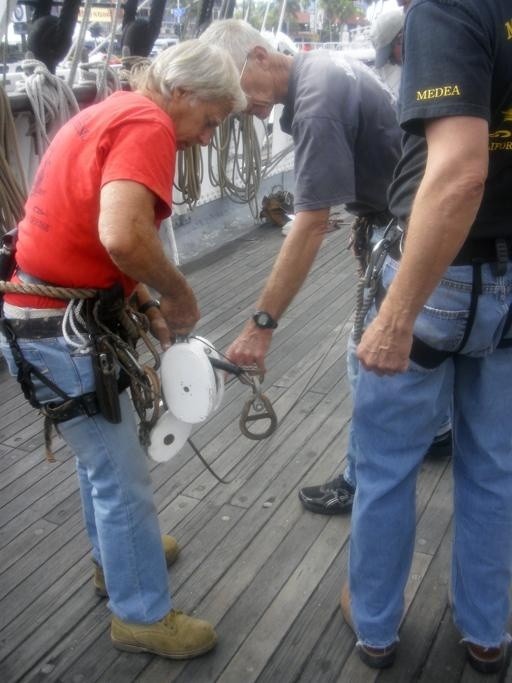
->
[237,55,247,86]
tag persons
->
[0,38,247,659]
[198,18,404,514]
[360,10,404,102]
[342,0,512,676]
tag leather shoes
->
[443,584,506,674]
[338,579,399,668]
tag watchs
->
[251,309,278,329]
[138,299,160,314]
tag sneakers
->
[298,473,356,514]
[110,610,218,660]
[89,531,178,598]
[423,428,454,458]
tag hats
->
[370,7,407,71]
